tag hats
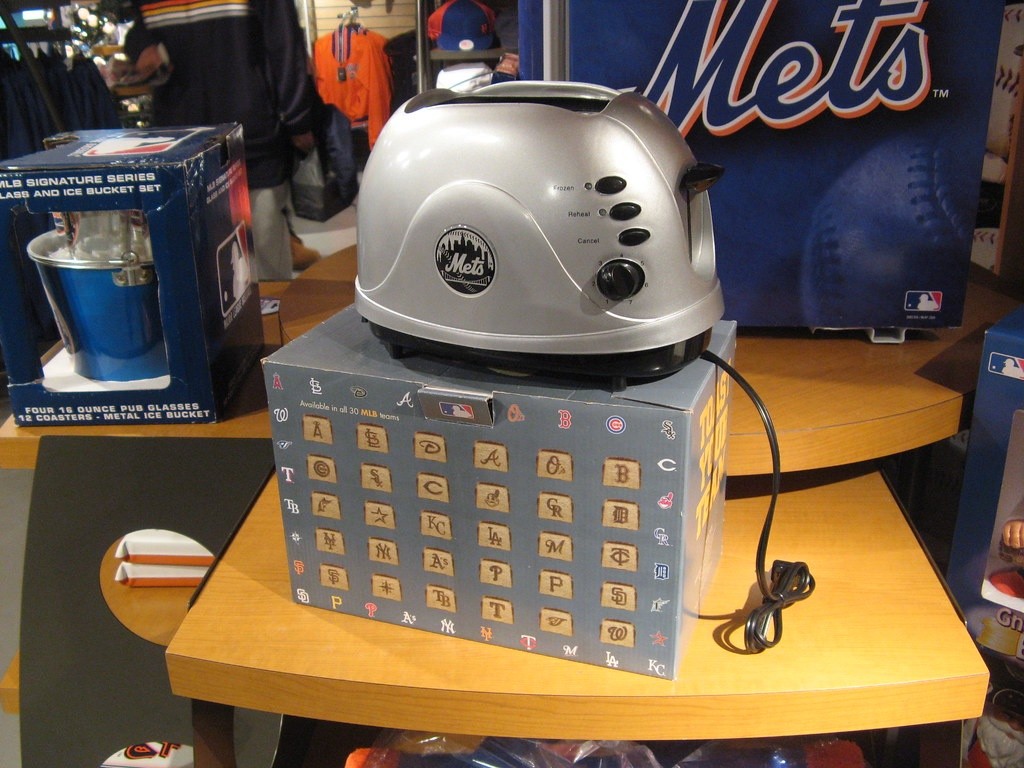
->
[429,0,496,50]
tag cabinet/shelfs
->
[416,0,518,95]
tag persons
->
[98,0,319,284]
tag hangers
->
[315,10,387,49]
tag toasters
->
[354,78,728,385]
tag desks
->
[0,241,990,768]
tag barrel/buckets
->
[26,228,170,380]
